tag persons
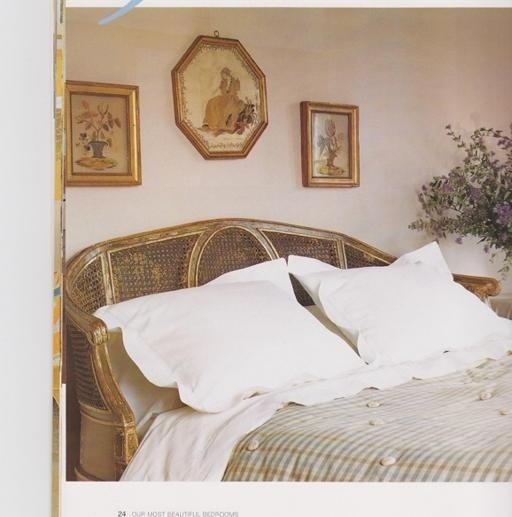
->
[320,118,342,169]
[198,67,244,137]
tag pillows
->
[107,305,361,442]
[288,241,512,388]
[92,258,382,414]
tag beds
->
[63,218,512,482]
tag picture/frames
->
[64,80,141,186]
[172,35,268,159]
[300,101,360,187]
